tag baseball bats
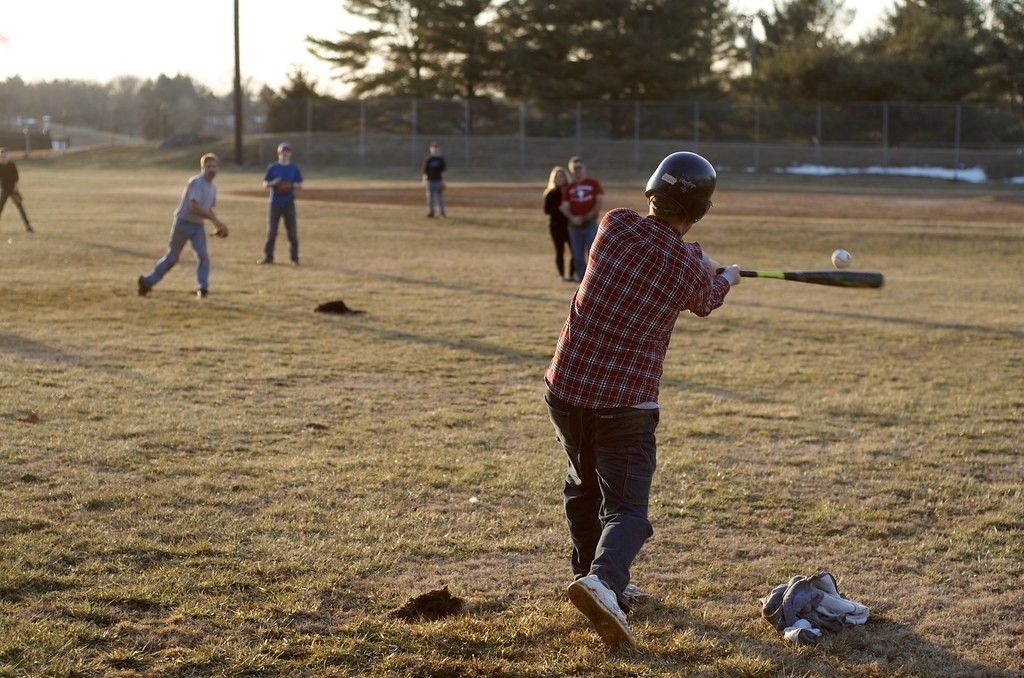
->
[716,267,882,289]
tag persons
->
[541,151,742,641]
[422,142,447,217]
[0,146,33,233]
[260,143,304,264]
[542,166,576,281]
[138,153,229,296]
[560,156,604,281]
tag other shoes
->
[443,214,446,219]
[137,276,150,297]
[427,213,434,217]
[196,290,207,300]
[26,227,34,233]
[289,259,298,267]
[256,256,272,264]
[560,277,564,281]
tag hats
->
[277,143,291,152]
[431,142,438,147]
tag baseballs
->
[831,249,852,269]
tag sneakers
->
[566,575,642,652]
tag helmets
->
[645,151,717,224]
